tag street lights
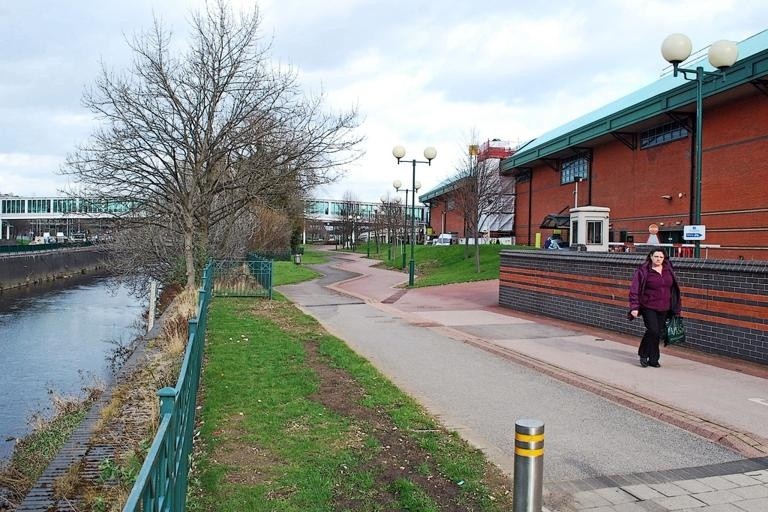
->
[373,180,401,270]
[661,33,739,258]
[393,146,437,287]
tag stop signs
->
[649,224,658,234]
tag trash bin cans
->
[294,254,301,265]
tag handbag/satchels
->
[663,316,686,344]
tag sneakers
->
[640,357,660,367]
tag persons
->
[495,239,500,244]
[626,249,682,368]
[608,242,635,253]
[482,231,489,244]
